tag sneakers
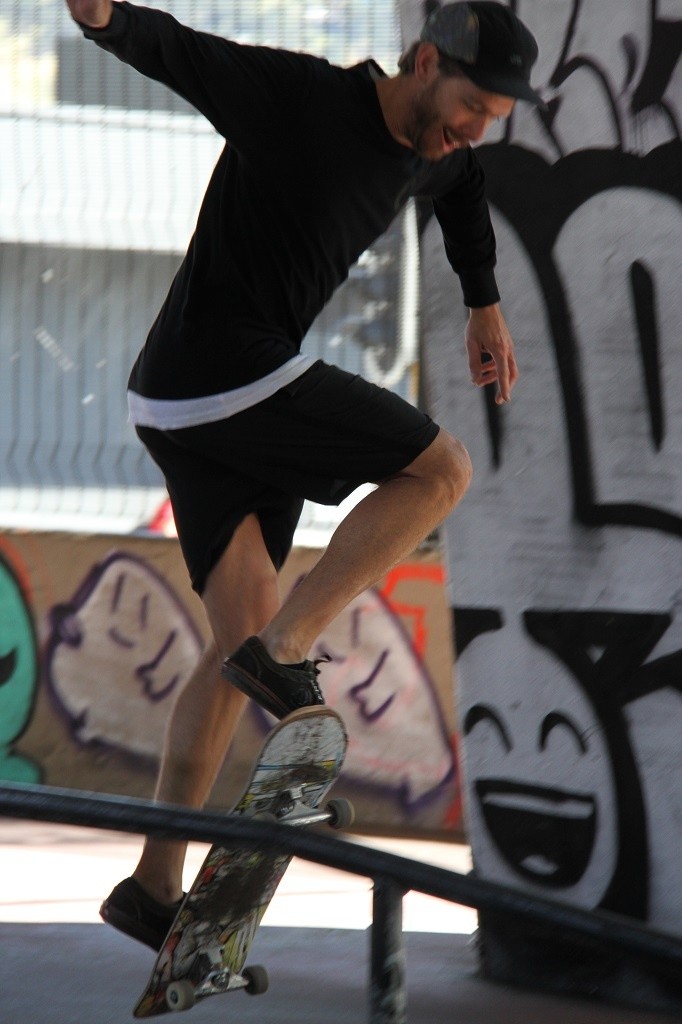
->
[98,877,186,954]
[221,634,331,721]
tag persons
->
[60,0,539,953]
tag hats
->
[420,0,546,112]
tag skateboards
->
[132,705,352,1020]
